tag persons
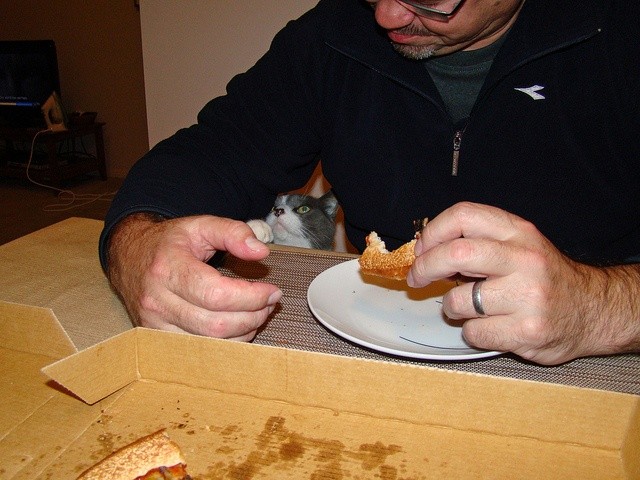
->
[100,0,639,368]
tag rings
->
[472,280,485,316]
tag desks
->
[0,121,107,196]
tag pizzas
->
[76,429,187,480]
[358,218,429,282]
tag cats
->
[245,189,341,252]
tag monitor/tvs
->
[0,39,62,107]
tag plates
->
[307,258,509,360]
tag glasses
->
[396,0,467,23]
